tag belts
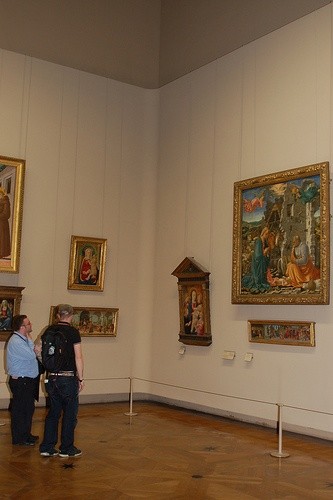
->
[47,372,74,376]
[10,376,32,380]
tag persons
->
[34,304,86,457]
[6,315,42,447]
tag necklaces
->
[14,333,27,342]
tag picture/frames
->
[0,155,26,275]
[0,285,26,342]
[66,235,108,292]
[49,306,119,337]
[231,160,330,306]
[246,319,316,348]
[171,256,213,347]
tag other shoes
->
[13,435,39,446]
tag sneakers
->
[59,446,82,457]
[40,448,59,456]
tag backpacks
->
[42,330,67,373]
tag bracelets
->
[78,379,85,382]
